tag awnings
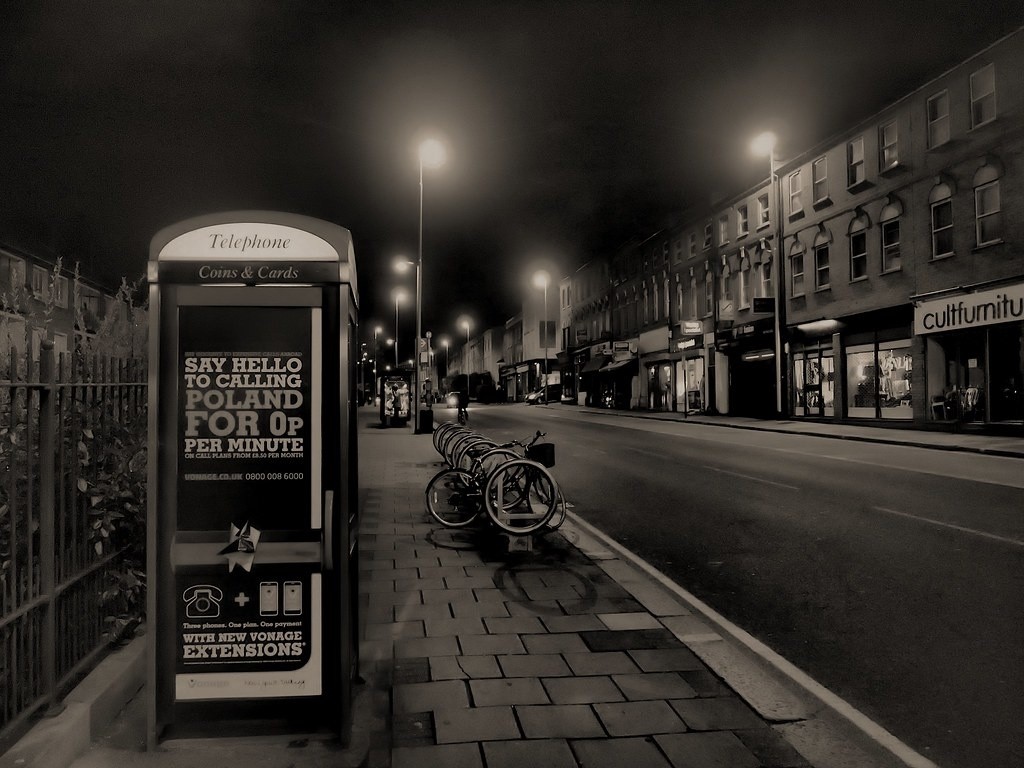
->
[582,362,601,371]
[600,360,628,371]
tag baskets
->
[525,443,555,468]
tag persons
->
[392,385,401,417]
[458,390,468,415]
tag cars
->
[525,383,562,404]
[444,392,460,408]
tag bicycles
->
[424,430,566,531]
[458,409,467,426]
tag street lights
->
[460,321,471,395]
[369,359,377,405]
[411,140,452,438]
[360,343,367,398]
[534,275,550,406]
[391,258,421,434]
[752,131,785,416]
[441,340,450,376]
[395,294,406,367]
[386,339,397,367]
[374,326,382,406]
